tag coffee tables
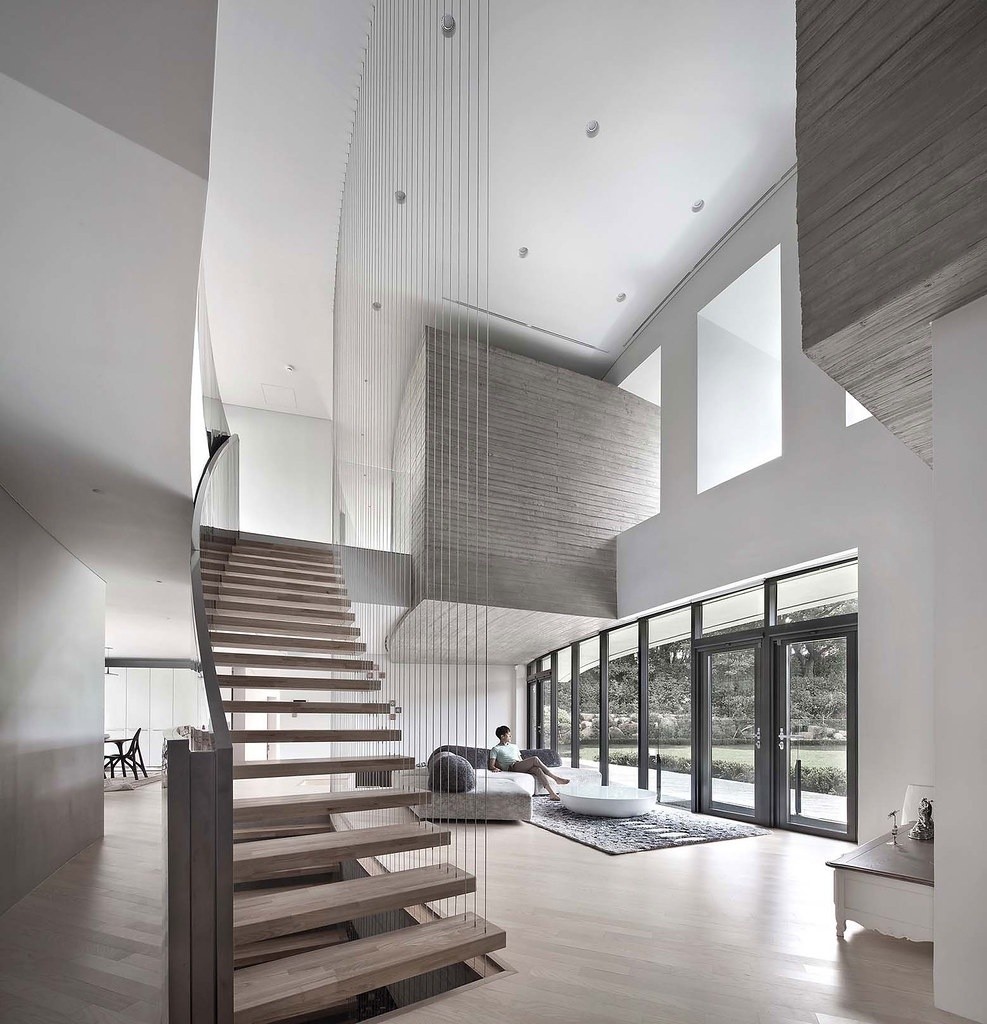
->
[559,785,658,818]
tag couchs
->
[401,745,602,820]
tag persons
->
[489,726,570,800]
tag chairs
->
[103,728,149,780]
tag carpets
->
[103,772,163,792]
[520,795,773,856]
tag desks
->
[104,739,133,776]
[825,821,934,943]
